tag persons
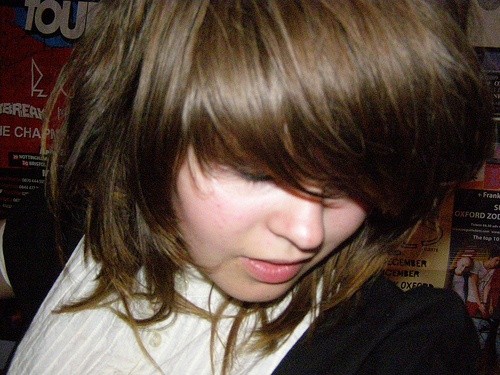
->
[448,257,473,305]
[0,0,499,374]
[467,249,500,322]
